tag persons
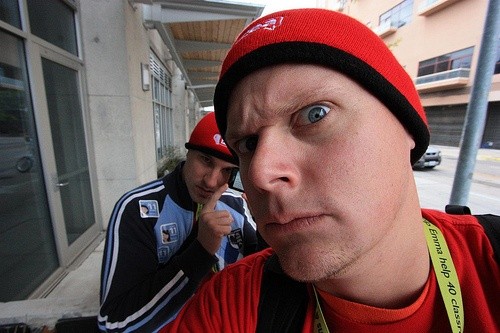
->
[98,110,256,333]
[156,9,500,333]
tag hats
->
[214,7,429,167]
[185,112,239,165]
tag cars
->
[412,146,441,172]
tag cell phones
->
[228,167,246,194]
